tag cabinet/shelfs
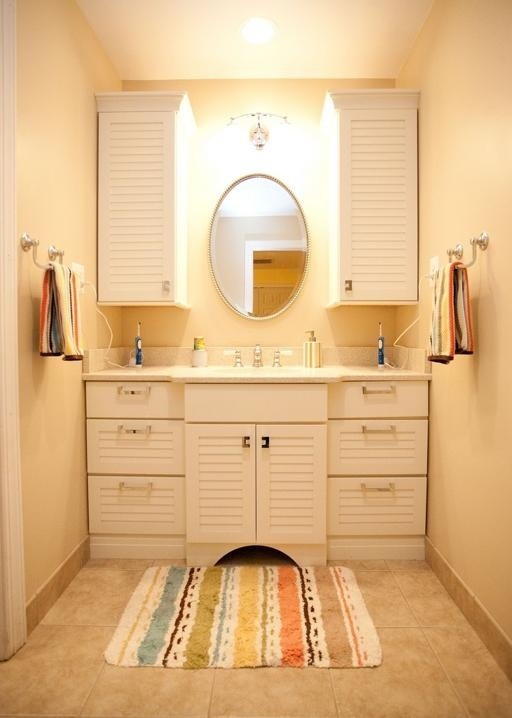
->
[82,380,185,560]
[98,91,194,308]
[184,380,327,568]
[328,380,427,559]
[323,90,420,309]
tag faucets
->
[252,344,265,367]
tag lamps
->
[243,109,286,150]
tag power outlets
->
[427,256,440,286]
[71,263,89,298]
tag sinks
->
[186,367,319,378]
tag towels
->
[427,261,475,364]
[37,262,88,363]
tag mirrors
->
[209,174,310,322]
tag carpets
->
[106,563,386,673]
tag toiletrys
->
[303,329,323,368]
